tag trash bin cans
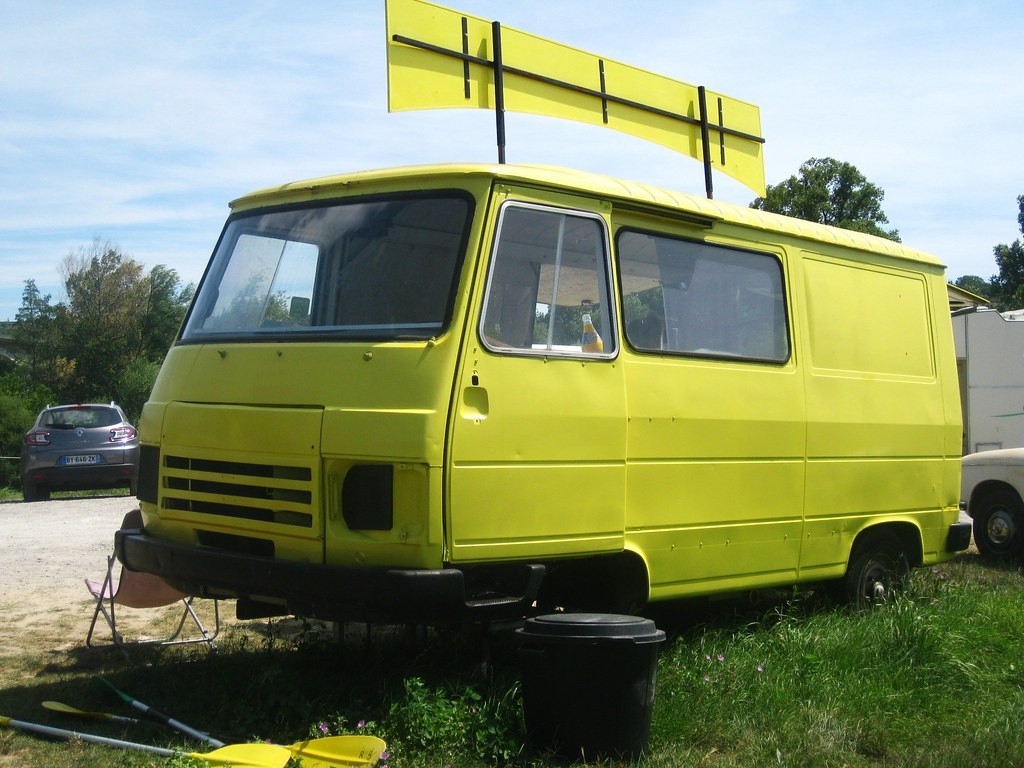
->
[512,611,667,763]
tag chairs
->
[86,506,220,668]
[627,316,680,350]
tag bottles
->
[582,313,603,354]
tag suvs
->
[20,401,138,500]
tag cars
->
[962,446,1024,559]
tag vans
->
[114,163,972,637]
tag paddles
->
[41,700,387,768]
[89,673,227,751]
[2,714,292,768]
